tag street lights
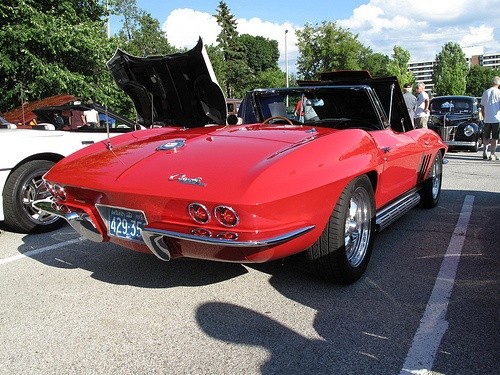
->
[284,29,290,108]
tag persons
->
[81,100,100,128]
[478,76,500,161]
[414,82,430,130]
[294,93,308,123]
[402,83,417,129]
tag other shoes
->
[483,153,489,160]
[490,154,500,160]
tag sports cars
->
[31,70,449,286]
[0,34,244,235]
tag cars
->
[427,95,484,152]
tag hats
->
[404,83,411,88]
